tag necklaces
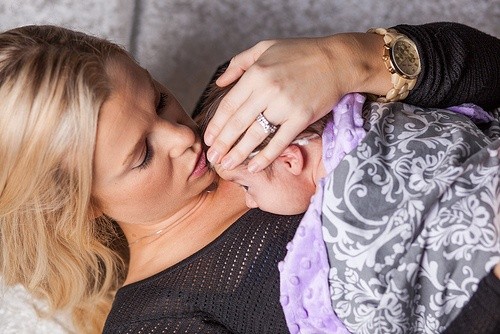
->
[128,181,219,249]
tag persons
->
[200,60,500,334]
[3,22,500,334]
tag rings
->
[258,113,278,136]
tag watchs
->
[366,27,424,104]
